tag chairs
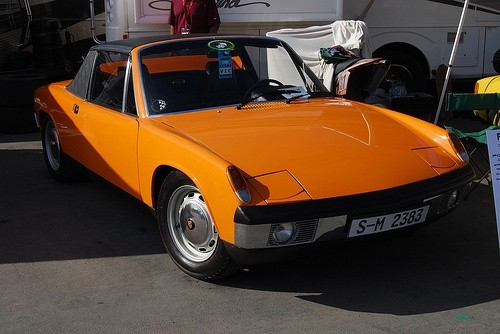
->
[443,93,499,200]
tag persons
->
[168,0,221,36]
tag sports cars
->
[30,31,477,281]
